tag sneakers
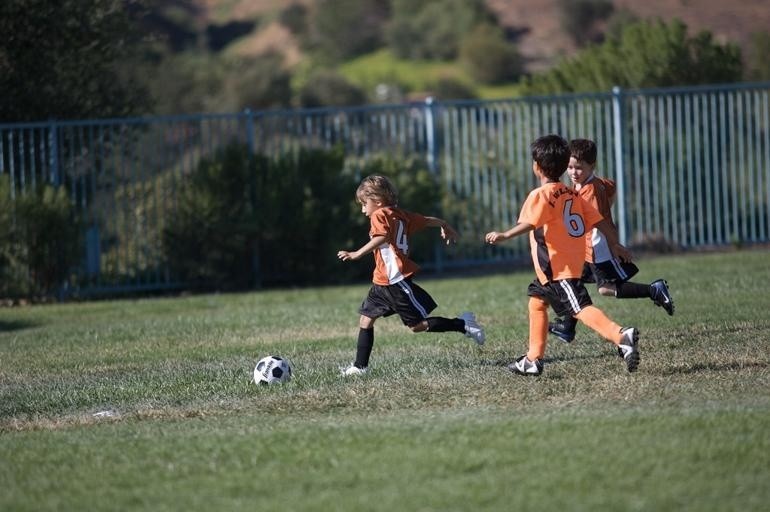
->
[336,363,368,379]
[651,278,678,318]
[617,326,642,373]
[546,321,576,342]
[507,356,543,376]
[458,311,487,346]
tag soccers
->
[253,355,290,387]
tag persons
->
[485,135,639,375]
[548,139,676,343]
[337,174,485,377]
